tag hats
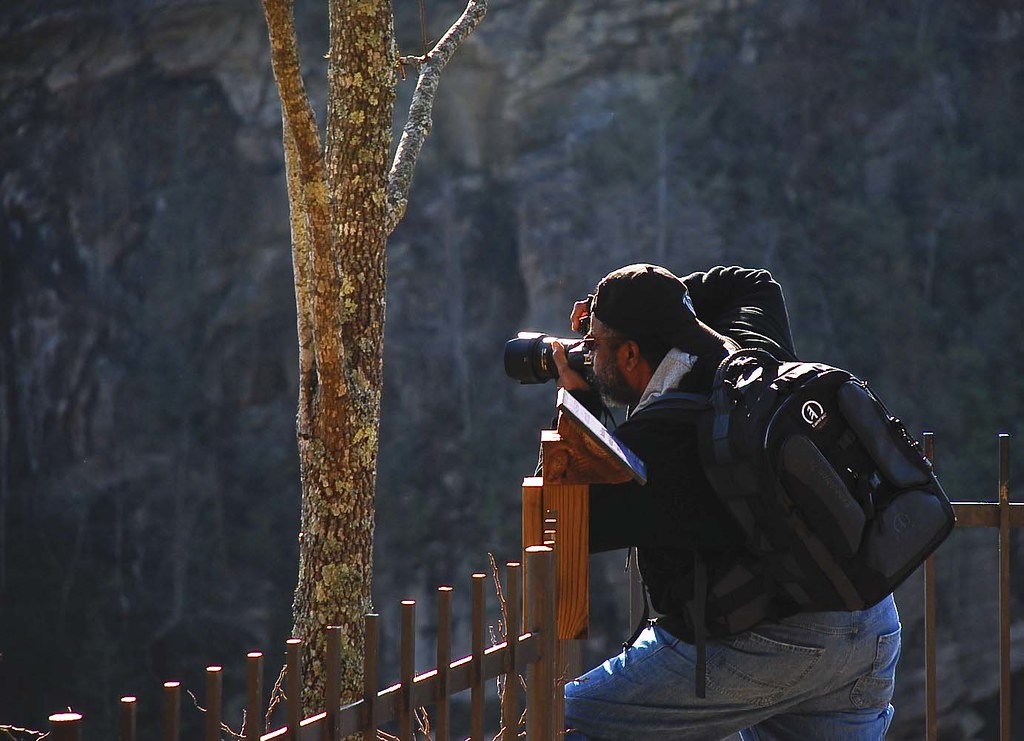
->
[590,263,726,355]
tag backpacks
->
[639,335,958,613]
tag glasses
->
[585,332,636,351]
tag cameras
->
[504,316,600,391]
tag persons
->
[551,264,901,741]
